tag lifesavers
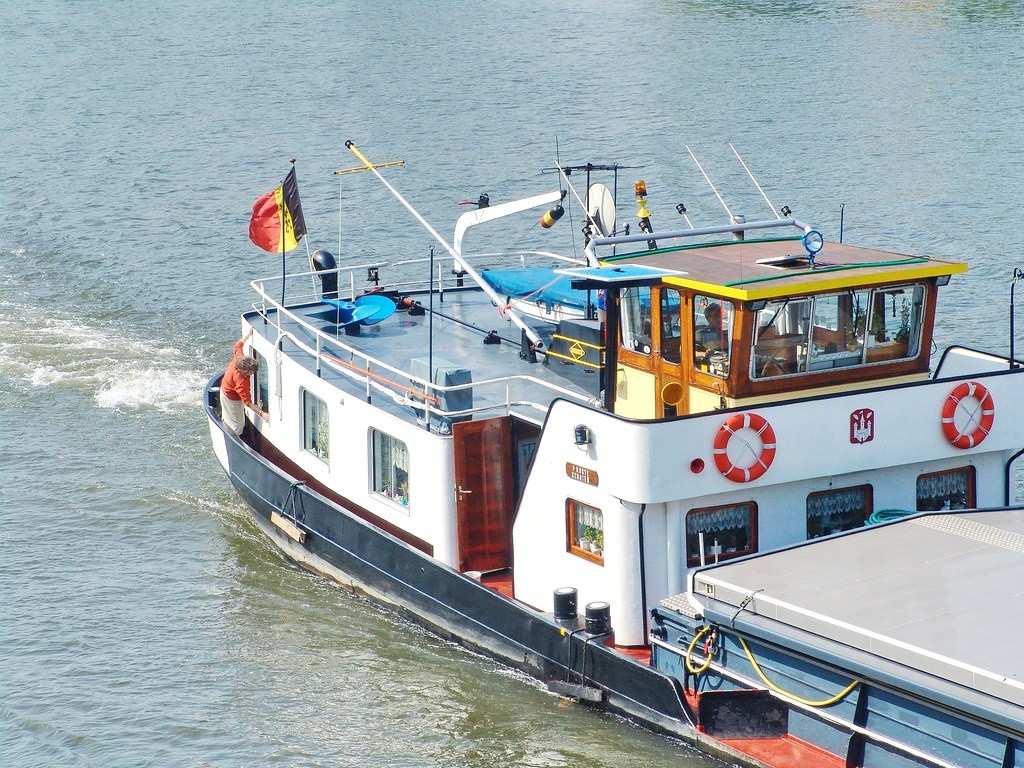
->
[941,381,995,449]
[712,412,777,482]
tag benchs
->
[662,323,802,361]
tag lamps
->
[801,231,824,269]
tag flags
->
[248,166,308,255]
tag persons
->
[219,327,269,439]
[694,302,729,376]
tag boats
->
[204,132,1024,767]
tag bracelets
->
[258,410,262,415]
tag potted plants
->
[844,321,857,351]
[579,526,604,557]
[894,298,910,344]
[400,481,408,505]
[854,305,884,349]
[383,476,398,497]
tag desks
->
[756,333,807,376]
[861,342,907,363]
[794,347,862,371]
